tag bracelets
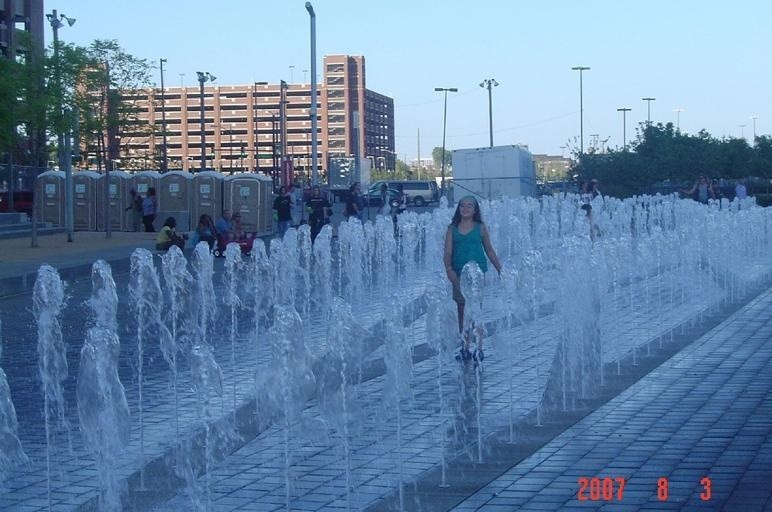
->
[446,267,452,272]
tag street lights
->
[197,71,217,171]
[254,81,268,172]
[435,88,459,195]
[159,59,171,171]
[268,110,279,190]
[672,110,684,126]
[479,78,498,145]
[45,8,77,244]
[643,98,655,124]
[210,123,234,175]
[739,117,759,140]
[617,109,631,150]
[570,67,591,153]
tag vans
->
[364,179,438,207]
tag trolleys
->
[207,215,248,258]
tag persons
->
[580,204,592,221]
[125,181,407,255]
[588,178,602,199]
[442,195,506,362]
[579,179,589,199]
[679,174,756,203]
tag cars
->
[0,190,33,218]
[363,187,408,208]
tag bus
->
[545,180,580,195]
